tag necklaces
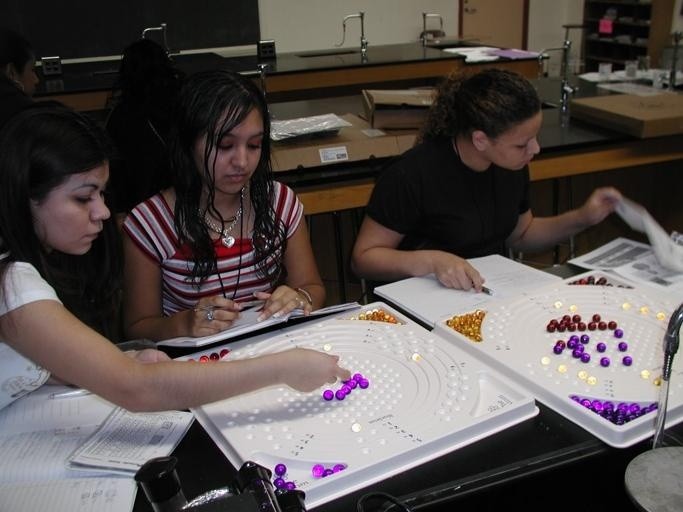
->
[196,186,246,250]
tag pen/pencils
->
[47,390,90,399]
[472,283,494,295]
[196,300,269,312]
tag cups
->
[639,55,650,74]
[624,60,639,89]
[599,64,612,87]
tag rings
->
[294,297,304,309]
[207,310,214,320]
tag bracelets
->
[295,286,313,305]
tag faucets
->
[657,301,683,381]
[256,65,269,109]
[539,39,574,115]
[422,11,444,46]
[140,23,166,49]
[340,10,368,56]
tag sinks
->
[293,48,359,58]
[425,41,504,52]
[385,427,682,512]
[540,99,557,112]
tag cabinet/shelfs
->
[582,0,676,69]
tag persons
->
[0,27,41,120]
[349,68,621,293]
[119,70,327,343]
[0,99,353,415]
[105,38,179,212]
